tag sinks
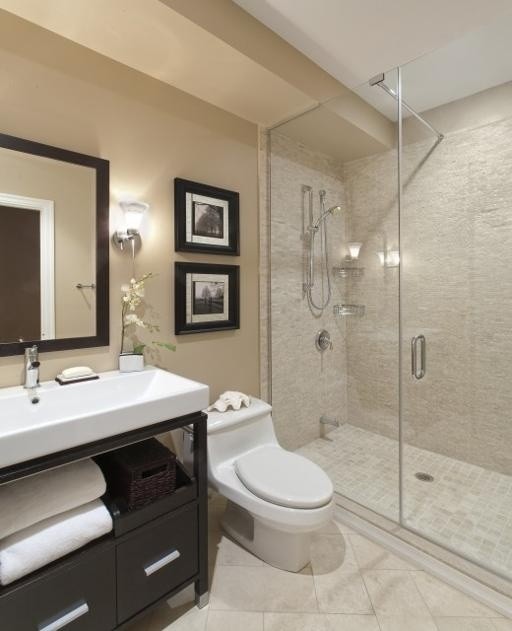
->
[0,363,210,468]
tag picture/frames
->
[175,262,240,335]
[173,177,240,256]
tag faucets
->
[23,345,40,388]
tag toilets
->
[169,393,335,573]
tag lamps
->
[113,201,150,263]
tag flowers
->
[120,272,176,354]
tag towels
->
[0,497,113,588]
[0,457,107,540]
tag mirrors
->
[0,132,109,357]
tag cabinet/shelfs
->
[0,409,210,631]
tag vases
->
[119,352,144,372]
